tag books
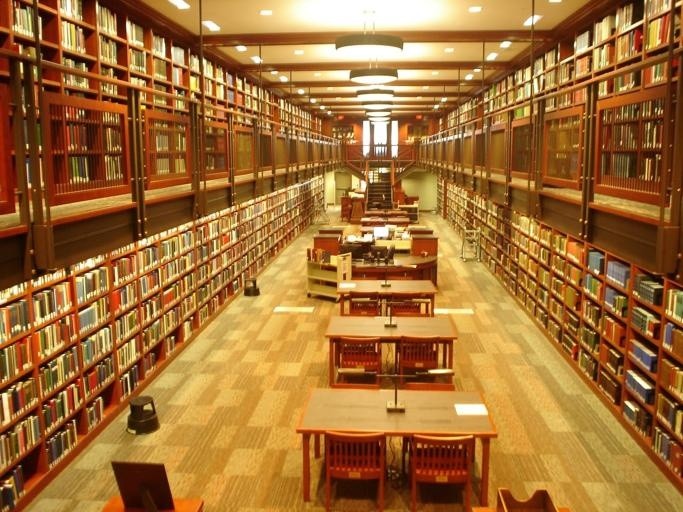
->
[0,175,324,511]
[1,0,322,186]
[438,179,683,479]
[438,0,683,182]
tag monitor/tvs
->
[371,246,387,262]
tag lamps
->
[333,30,404,127]
